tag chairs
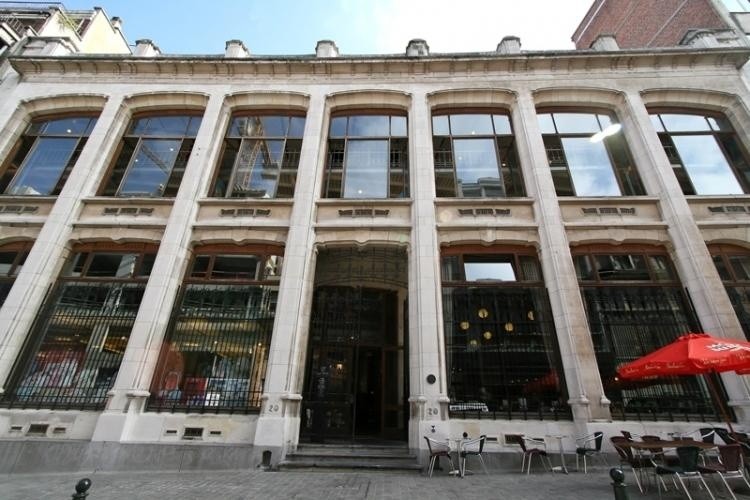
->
[610,427,750,500]
[515,430,603,475]
[423,434,488,479]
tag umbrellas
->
[618,332,750,444]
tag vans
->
[625,395,716,413]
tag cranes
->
[237,117,280,187]
